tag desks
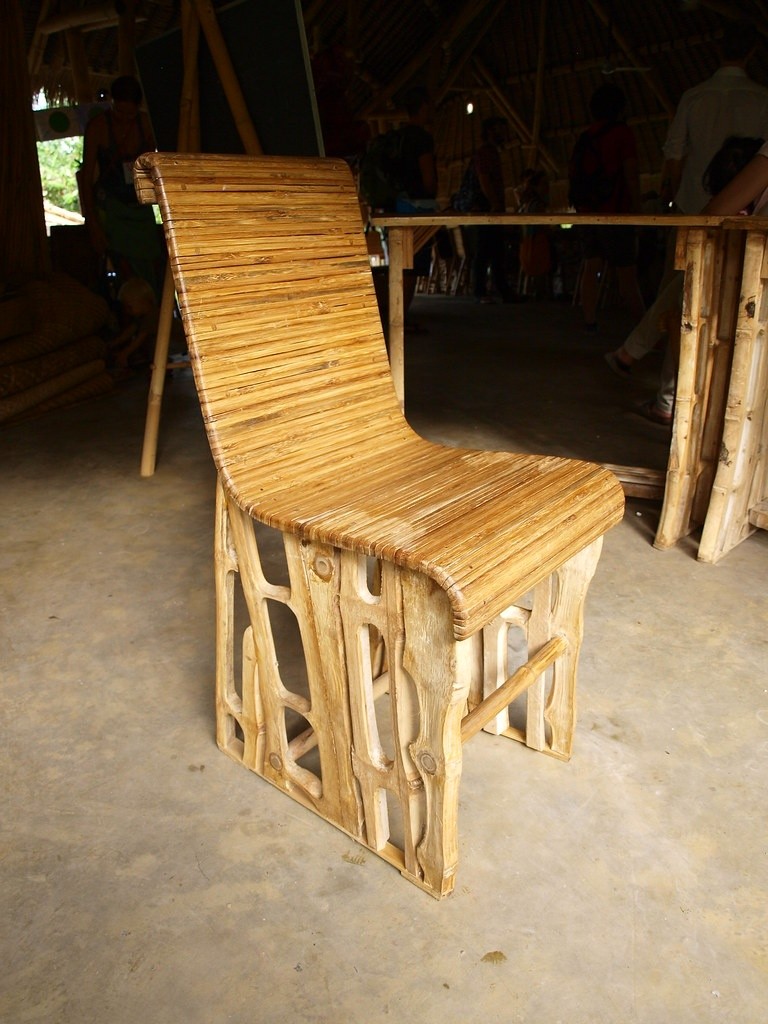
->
[370,213,768,565]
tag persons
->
[76,75,163,276]
[375,86,438,334]
[421,90,655,345]
[106,277,173,377]
[605,18,768,425]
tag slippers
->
[638,398,673,425]
[604,351,632,381]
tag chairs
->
[131,151,624,901]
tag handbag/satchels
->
[702,134,766,215]
